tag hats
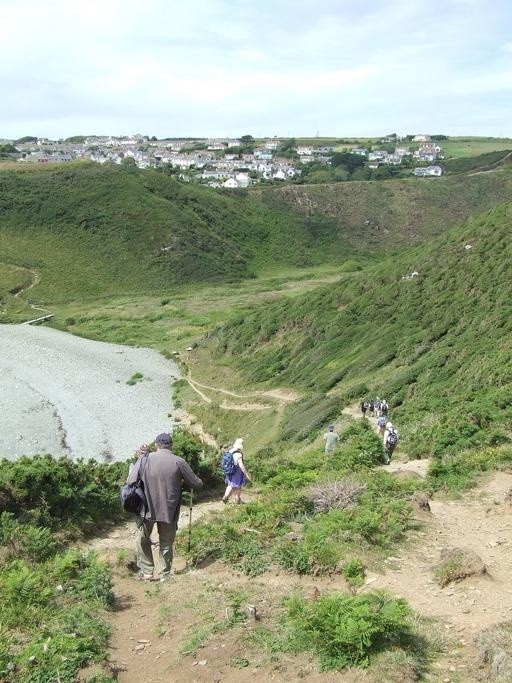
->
[233,438,243,449]
[328,425,334,430]
[156,433,172,444]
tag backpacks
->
[222,449,243,475]
[385,428,397,450]
[379,417,387,427]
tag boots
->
[221,496,229,504]
[235,498,245,504]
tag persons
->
[125,432,204,581]
[219,437,252,504]
[360,395,398,464]
[322,424,340,454]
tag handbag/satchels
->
[120,481,143,513]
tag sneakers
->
[385,459,390,464]
[138,570,154,579]
